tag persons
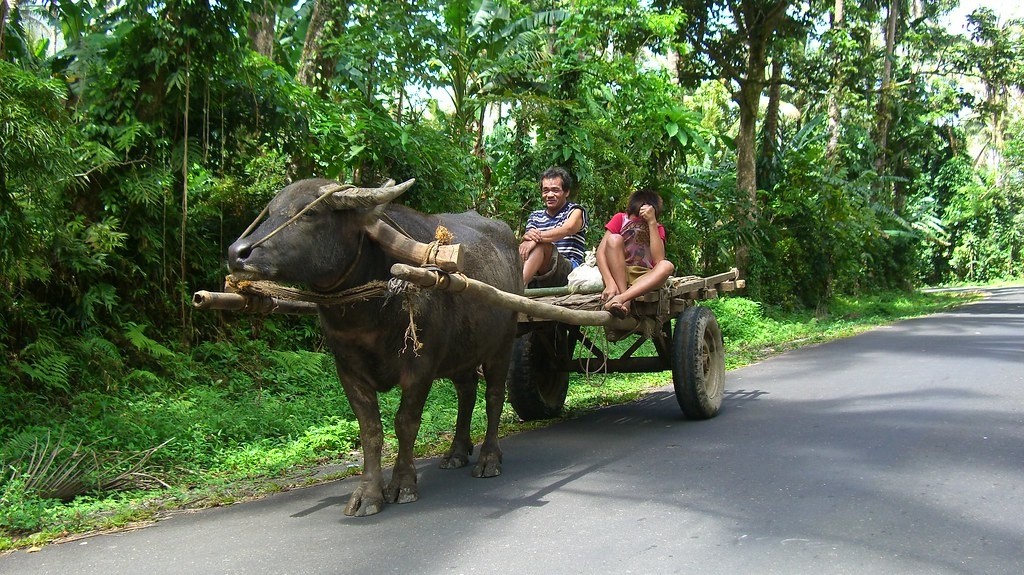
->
[596,190,674,320]
[519,167,587,289]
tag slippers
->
[606,302,629,320]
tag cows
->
[225,175,527,520]
